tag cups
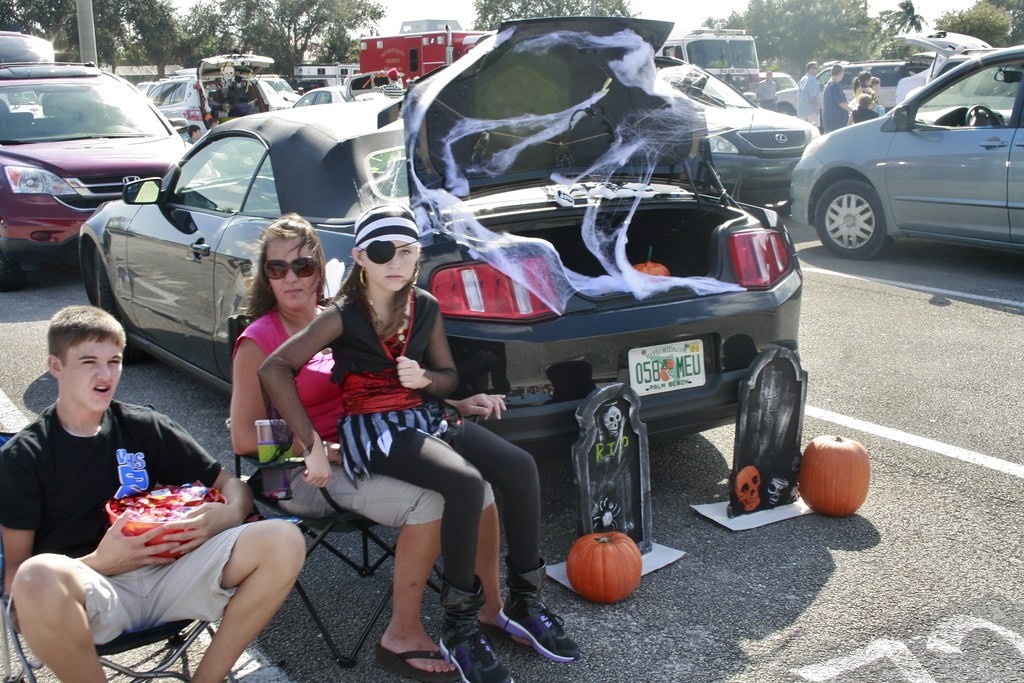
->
[254,419,290,491]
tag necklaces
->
[367,289,413,347]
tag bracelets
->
[323,441,328,457]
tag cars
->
[646,54,822,205]
[758,71,802,118]
[789,46,1024,263]
[893,25,1018,114]
[0,60,197,292]
[131,23,491,169]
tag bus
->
[657,27,761,101]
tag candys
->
[111,480,224,524]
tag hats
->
[387,67,398,79]
[354,204,419,252]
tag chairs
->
[220,310,456,670]
[0,427,235,682]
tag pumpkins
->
[799,434,869,516]
[631,260,670,277]
[566,531,642,602]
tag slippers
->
[376,644,462,683]
[479,622,533,647]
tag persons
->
[756,71,776,112]
[208,118,219,129]
[822,64,854,135]
[258,203,580,683]
[847,71,885,126]
[796,62,820,127]
[188,125,201,144]
[370,68,404,99]
[230,212,507,683]
[0,305,305,683]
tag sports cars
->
[77,9,806,461]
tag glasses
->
[264,257,319,280]
[812,66,818,69]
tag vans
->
[0,31,57,121]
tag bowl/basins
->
[104,494,228,559]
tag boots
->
[496,555,581,663]
[440,573,514,683]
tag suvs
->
[772,60,909,124]
